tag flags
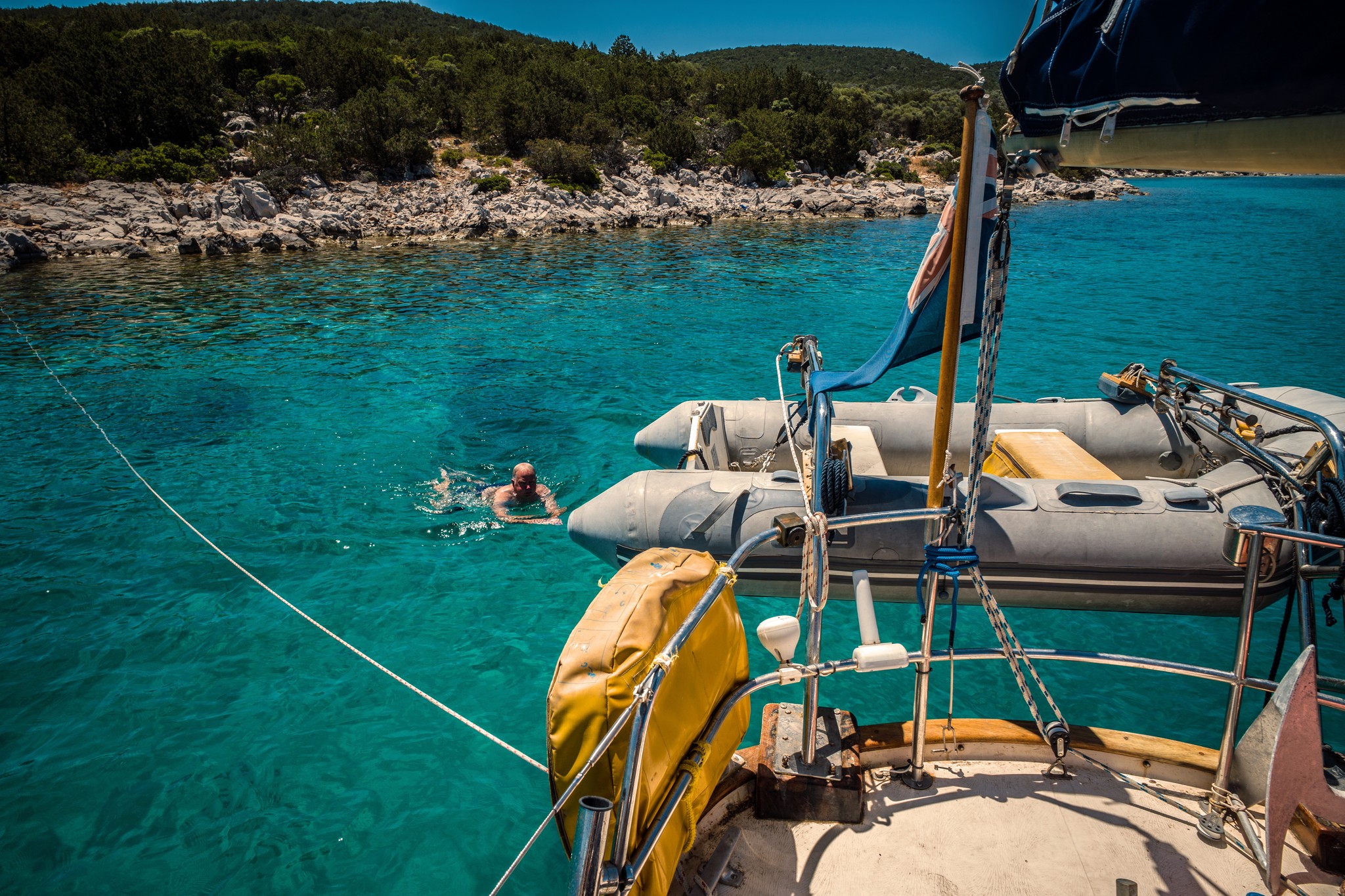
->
[808,109,1001,439]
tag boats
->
[563,353,1345,622]
[540,60,1345,896]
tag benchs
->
[830,424,888,476]
[983,429,1122,480]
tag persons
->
[428,463,569,525]
